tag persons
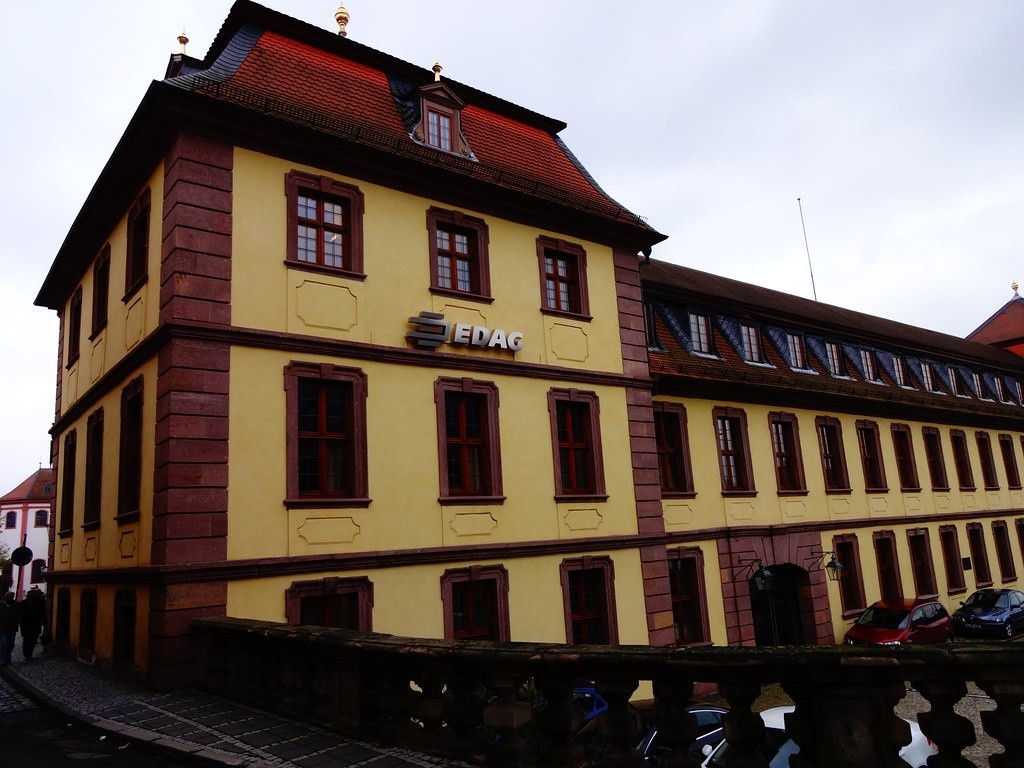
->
[0,592,22,666]
[19,590,45,660]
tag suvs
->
[839,595,954,645]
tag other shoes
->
[0,656,11,665]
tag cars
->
[630,696,731,763]
[700,705,936,768]
[952,587,1024,641]
[491,682,611,746]
